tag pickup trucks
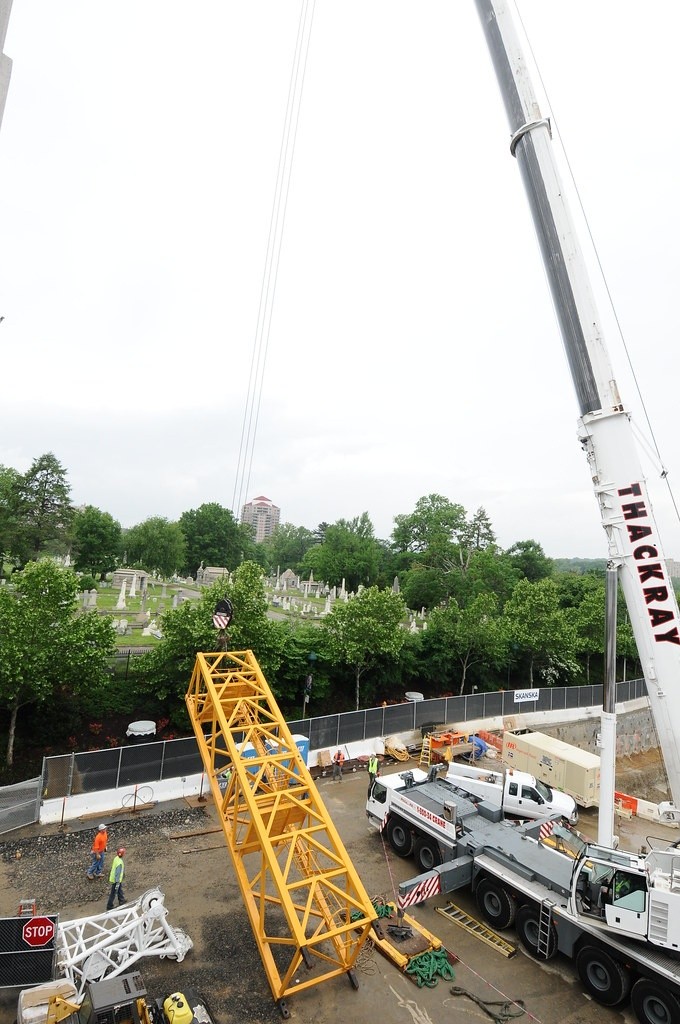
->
[448,759,579,824]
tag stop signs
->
[22,916,55,947]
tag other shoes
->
[96,873,105,876]
[87,872,93,879]
[107,906,118,910]
[119,900,127,905]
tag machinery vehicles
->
[368,2,680,1024]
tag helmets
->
[118,847,126,856]
[620,872,626,876]
[372,752,376,756]
[99,824,107,830]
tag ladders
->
[433,899,517,958]
[420,734,431,767]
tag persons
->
[333,748,345,780]
[107,848,127,911]
[367,754,379,784]
[616,875,631,896]
[86,824,109,879]
